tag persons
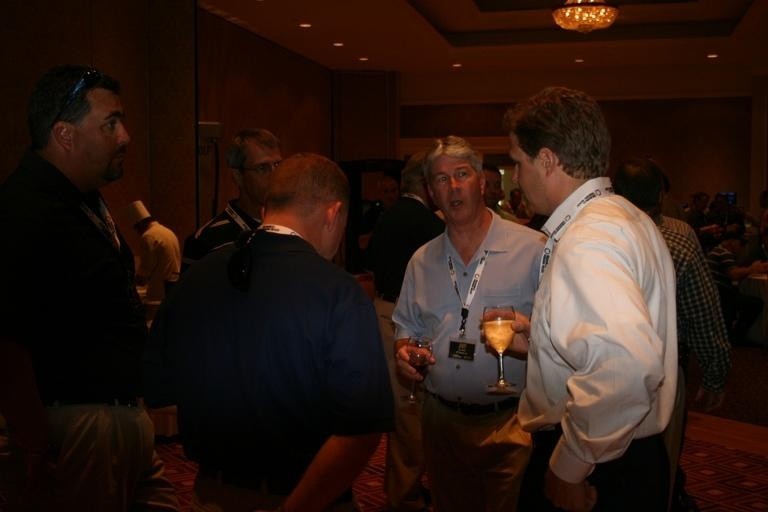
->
[499,187,527,218]
[704,231,768,347]
[147,149,398,508]
[681,188,768,289]
[476,81,682,511]
[351,172,403,237]
[390,131,553,511]
[478,161,520,226]
[629,156,735,512]
[357,150,445,511]
[122,197,181,302]
[180,127,282,275]
[2,62,180,511]
[608,156,664,220]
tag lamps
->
[552,1,619,35]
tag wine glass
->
[482,304,518,388]
[400,335,434,401]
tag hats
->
[128,201,151,227]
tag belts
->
[431,393,520,416]
